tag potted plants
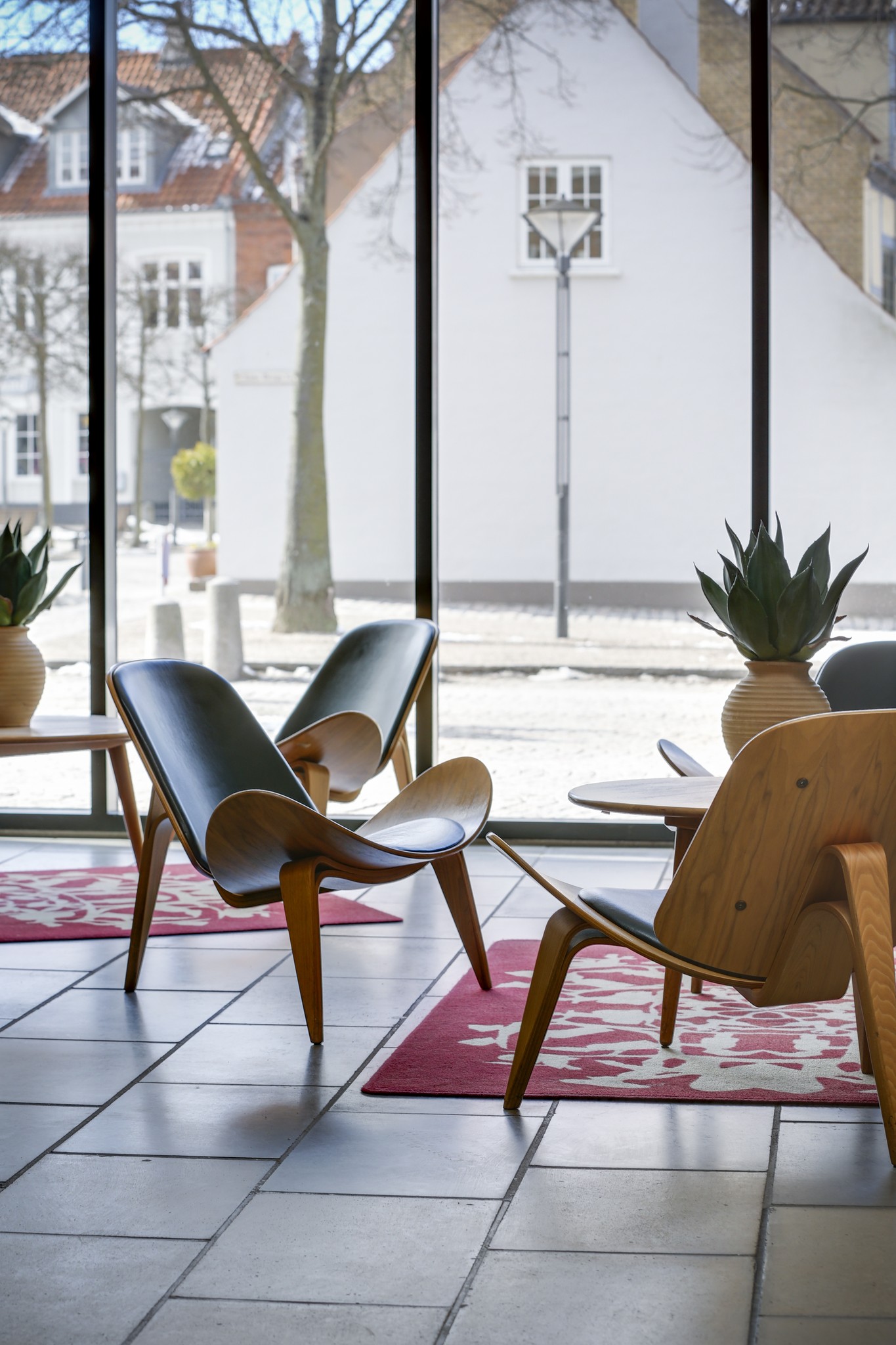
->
[170,443,215,576]
[0,518,85,727]
[686,511,868,759]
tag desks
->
[0,716,144,870]
[567,775,723,1047]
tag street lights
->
[519,194,604,640]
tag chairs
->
[106,658,493,1044]
[656,640,896,776]
[486,708,896,1166]
[125,617,440,994]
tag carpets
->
[361,939,879,1106]
[0,865,404,942]
